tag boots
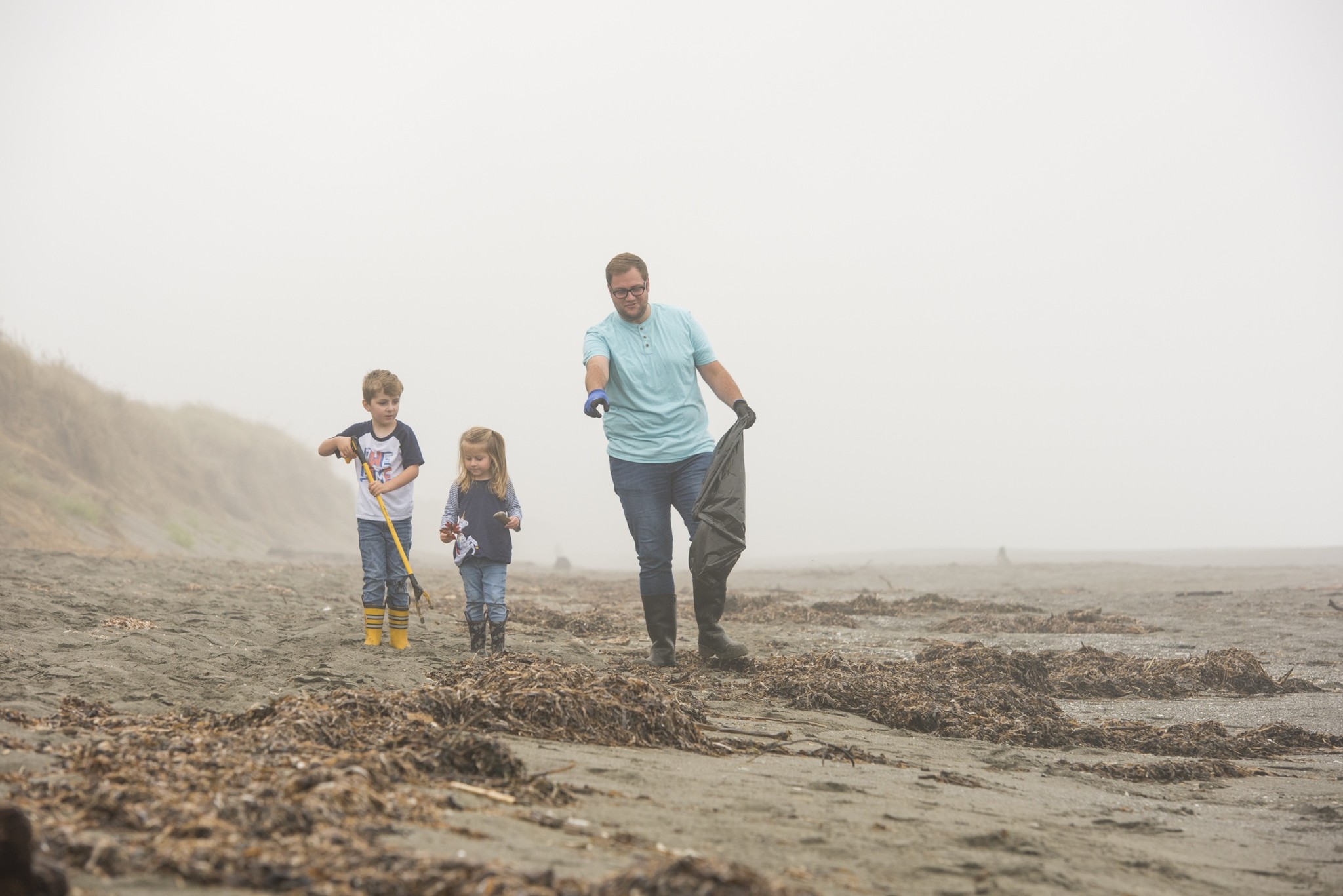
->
[386,596,412,649]
[361,595,386,645]
[632,593,678,667]
[693,585,749,659]
[464,610,489,654]
[483,609,510,654]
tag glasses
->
[609,280,646,299]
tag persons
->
[584,253,756,666]
[440,427,523,659]
[318,368,422,651]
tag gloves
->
[732,399,757,430]
[583,389,610,418]
